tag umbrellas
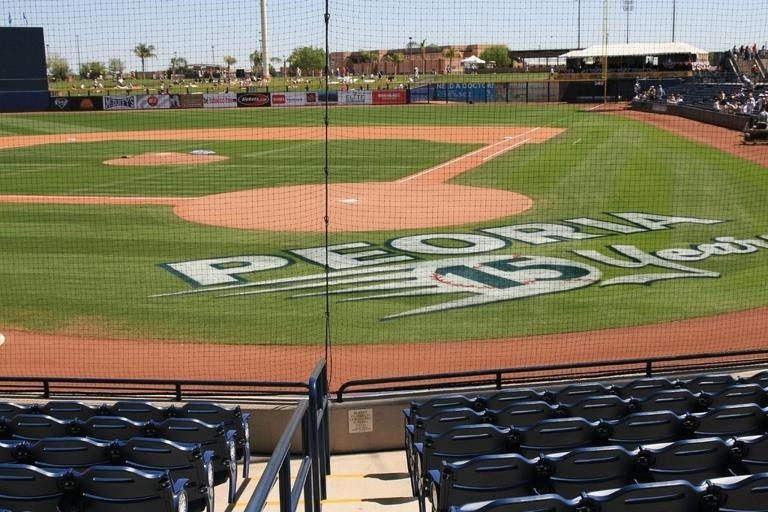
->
[459,53,487,66]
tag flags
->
[8,12,12,25]
[23,13,28,24]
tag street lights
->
[211,44,215,66]
[46,44,51,75]
[623,0,635,44]
[408,36,413,75]
[74,34,82,76]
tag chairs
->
[0,399,252,511]
[401,367,768,512]
[639,83,768,116]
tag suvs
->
[374,70,384,75]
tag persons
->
[740,117,758,145]
[548,42,767,130]
[92,64,497,92]
